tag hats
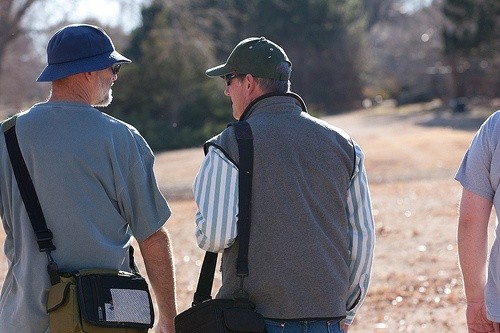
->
[35,23,133,84]
[204,35,292,83]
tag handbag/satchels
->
[44,266,157,333]
[172,294,268,333]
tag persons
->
[193,36,375,333]
[0,24,176,333]
[455,111,500,333]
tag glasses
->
[108,62,121,76]
[220,72,249,85]
